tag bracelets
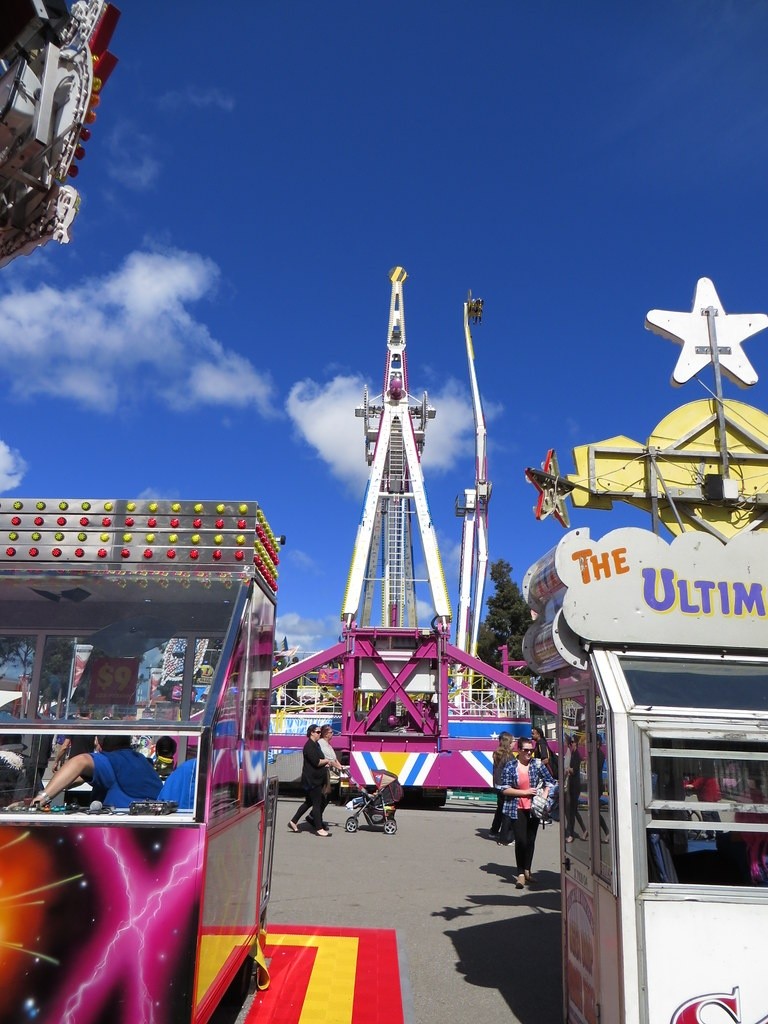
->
[38,790,52,804]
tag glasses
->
[571,741,577,743]
[522,749,535,753]
[314,731,321,734]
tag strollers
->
[328,766,404,837]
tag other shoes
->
[700,833,713,840]
[564,835,575,843]
[581,830,588,840]
[604,834,610,843]
[313,827,332,837]
[288,821,302,833]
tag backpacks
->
[547,750,559,778]
[531,780,552,829]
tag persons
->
[30,735,163,807]
[597,734,609,843]
[488,726,559,888]
[287,724,348,838]
[52,713,110,773]
[685,777,723,840]
[564,733,589,843]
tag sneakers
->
[305,815,330,830]
[488,831,501,838]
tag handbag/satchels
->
[321,769,332,795]
[500,813,515,843]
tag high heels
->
[516,875,526,889]
[525,869,538,883]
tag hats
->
[573,734,581,743]
[532,727,543,737]
